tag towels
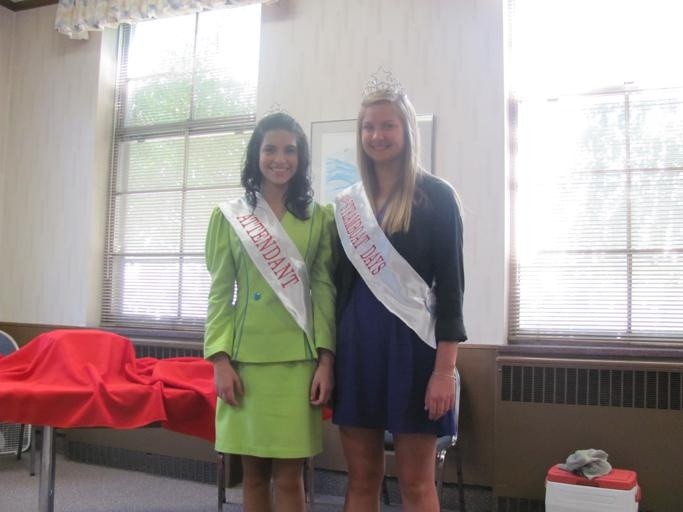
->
[557,448,614,480]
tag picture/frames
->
[306,113,437,205]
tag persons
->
[325,91,468,512]
[204,112,338,511]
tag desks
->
[0,356,238,511]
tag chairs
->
[382,363,468,512]
[0,330,36,476]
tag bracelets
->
[432,370,456,382]
